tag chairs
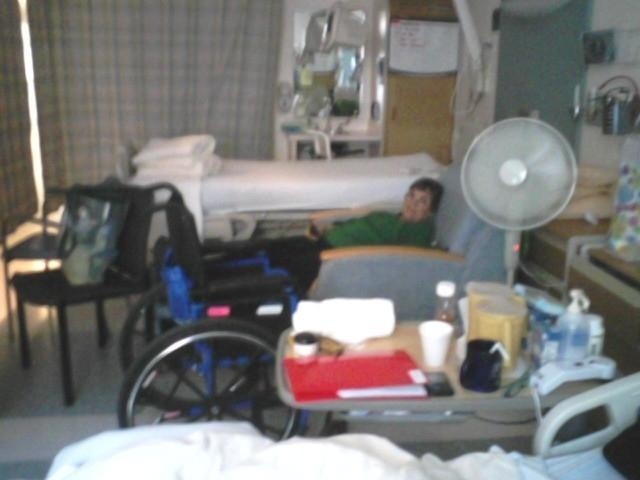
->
[1,176,153,407]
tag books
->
[282,350,430,406]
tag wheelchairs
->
[117,182,302,441]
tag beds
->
[44,372,640,480]
[116,138,446,242]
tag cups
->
[419,321,452,366]
[458,281,529,393]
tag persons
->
[202,176,444,279]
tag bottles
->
[522,297,560,368]
[588,313,604,357]
[434,280,456,325]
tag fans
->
[460,117,578,284]
